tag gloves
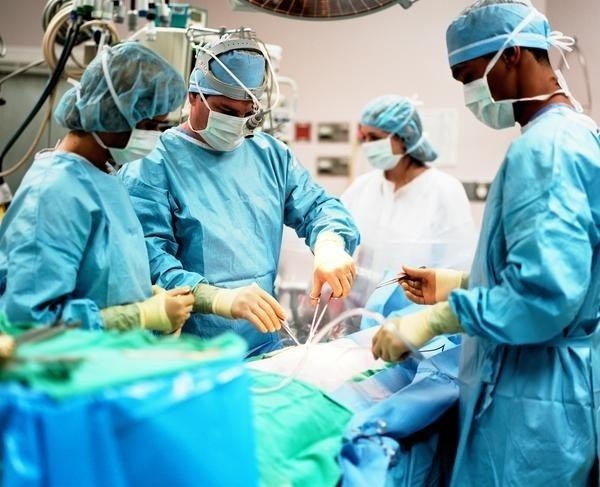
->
[372,300,458,362]
[213,283,286,334]
[310,230,358,308]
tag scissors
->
[305,291,343,343]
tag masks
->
[184,92,253,154]
[461,35,527,130]
[359,123,418,173]
[79,89,161,168]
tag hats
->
[443,1,551,66]
[188,51,269,102]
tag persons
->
[1,38,197,345]
[366,0,597,486]
[332,92,474,319]
[113,39,365,342]
